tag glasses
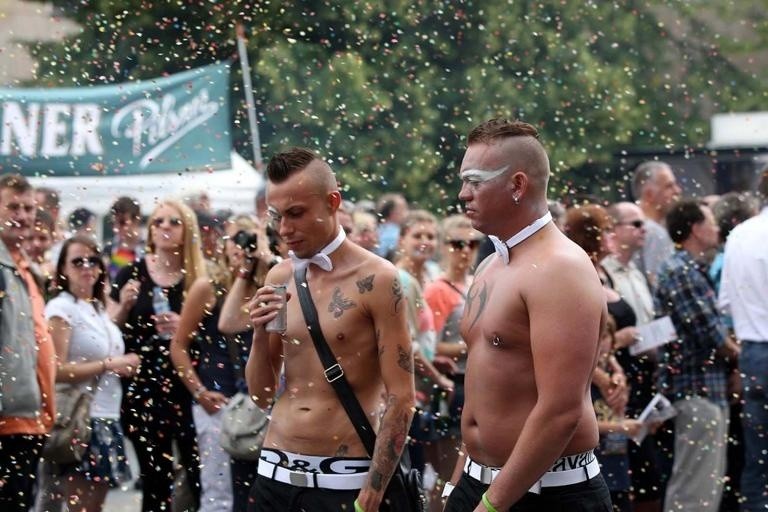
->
[446,239,482,248]
[152,217,183,227]
[67,257,101,268]
[617,221,643,228]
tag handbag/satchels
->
[387,441,431,511]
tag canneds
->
[264,285,287,333]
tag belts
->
[257,457,369,490]
[464,454,601,495]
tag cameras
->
[233,226,281,260]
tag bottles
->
[153,284,176,341]
